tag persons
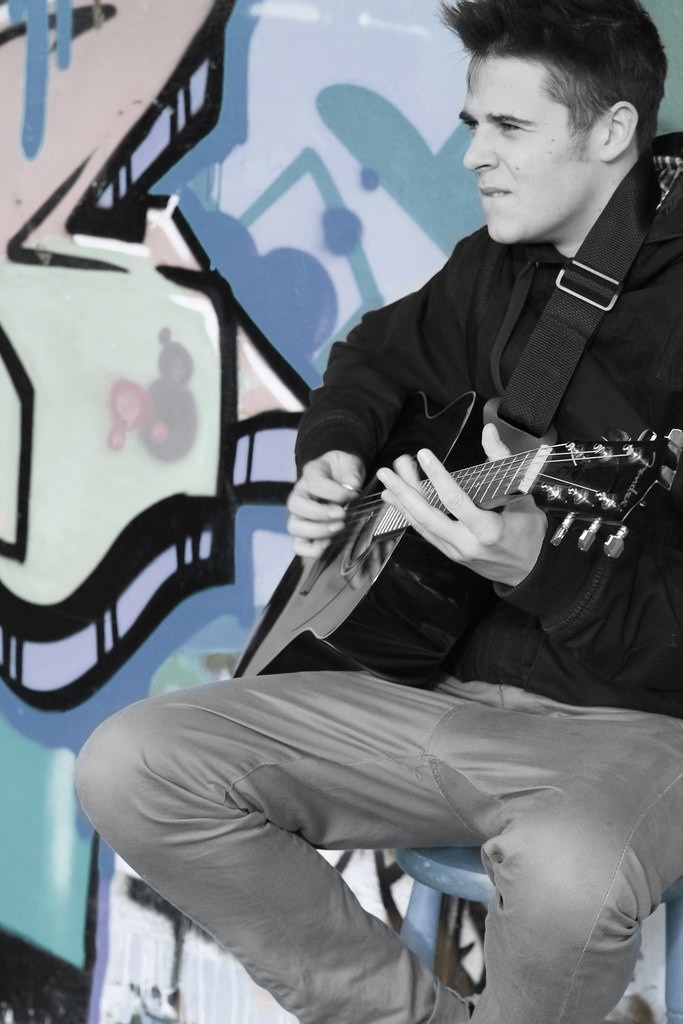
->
[73,1,683,1024]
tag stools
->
[390,844,683,1024]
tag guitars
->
[234,391,683,686]
